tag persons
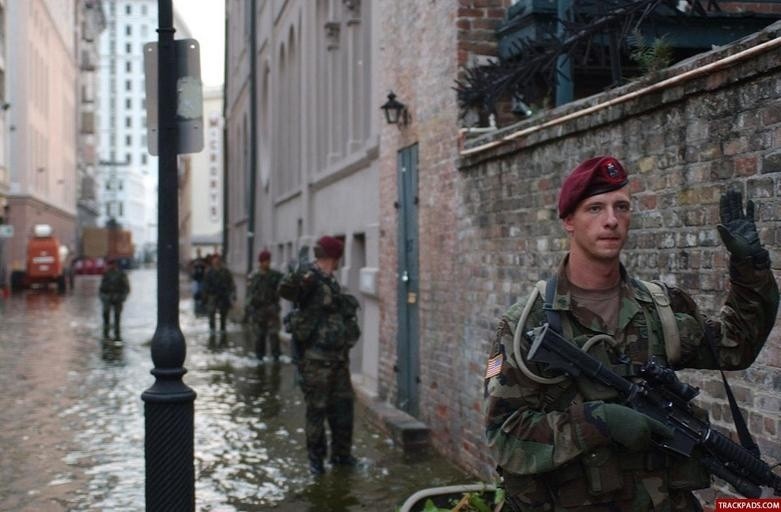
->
[189,248,237,331]
[481,156,780,512]
[98,258,129,341]
[276,237,364,475]
[242,251,285,362]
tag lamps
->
[380,90,408,127]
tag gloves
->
[605,405,673,457]
[717,189,771,254]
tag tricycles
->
[15,223,76,297]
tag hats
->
[259,251,270,261]
[318,235,340,257]
[559,157,628,217]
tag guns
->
[526,322,781,499]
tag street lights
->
[140,0,197,479]
